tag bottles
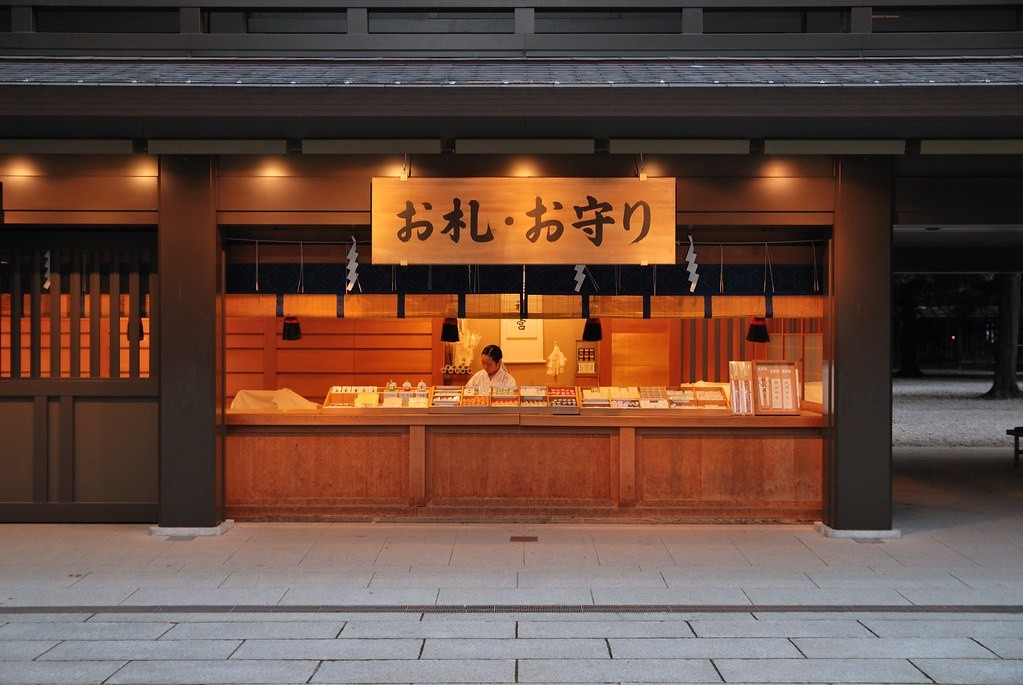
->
[386,379,427,392]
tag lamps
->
[747,316,770,342]
[126,316,144,340]
[582,296,603,341]
[441,296,460,342]
[281,314,301,341]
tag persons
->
[465,344,517,387]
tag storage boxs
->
[321,384,731,416]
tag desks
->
[1006,429,1023,462]
